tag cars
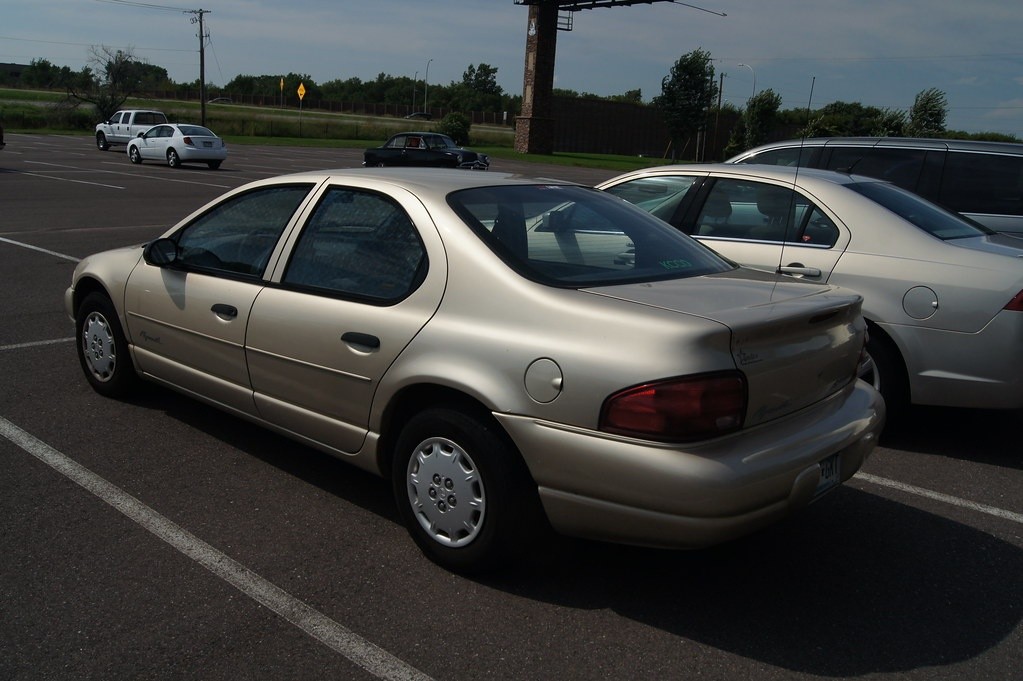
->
[404,111,433,121]
[476,162,1022,457]
[127,123,228,169]
[361,133,491,172]
[60,167,886,567]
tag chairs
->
[695,189,734,238]
[747,189,792,242]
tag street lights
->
[424,58,435,114]
[738,62,756,101]
[412,70,419,115]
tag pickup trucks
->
[95,109,169,151]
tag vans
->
[630,134,1023,256]
